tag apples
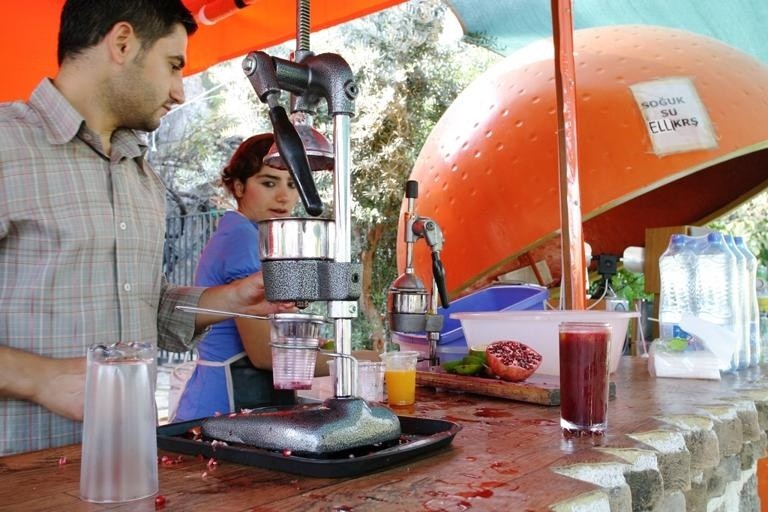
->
[318,337,327,348]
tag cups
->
[79,340,159,504]
[378,350,421,406]
[559,320,611,441]
[270,313,323,390]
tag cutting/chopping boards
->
[413,363,618,408]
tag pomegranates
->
[486,340,542,382]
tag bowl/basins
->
[450,309,642,376]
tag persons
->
[170,133,381,423]
[0,0,299,458]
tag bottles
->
[723,234,760,369]
[659,234,696,340]
[694,230,740,375]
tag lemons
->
[323,341,334,350]
[441,346,488,375]
